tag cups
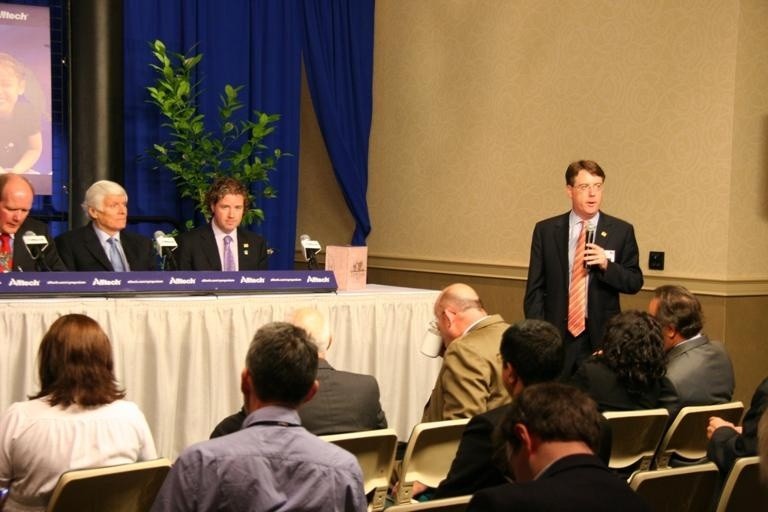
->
[419,321,443,358]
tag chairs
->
[47,458,170,512]
[385,494,473,512]
[602,409,669,470]
[653,401,745,470]
[627,463,718,512]
[318,429,398,512]
[718,456,759,512]
[387,418,471,505]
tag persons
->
[706,379,768,483]
[152,322,365,511]
[210,303,390,439]
[0,173,69,273]
[391,320,610,502]
[389,283,517,501]
[522,161,644,387]
[166,178,271,272]
[53,180,156,272]
[465,381,643,511]
[572,312,664,415]
[1,313,156,512]
[650,285,736,410]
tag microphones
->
[583,222,595,271]
[22,231,52,271]
[154,229,181,270]
[298,234,323,271]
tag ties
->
[566,220,588,338]
[106,237,126,274]
[0,234,14,274]
[223,236,237,272]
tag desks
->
[1,280,442,461]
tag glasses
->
[575,182,604,192]
[428,308,458,329]
